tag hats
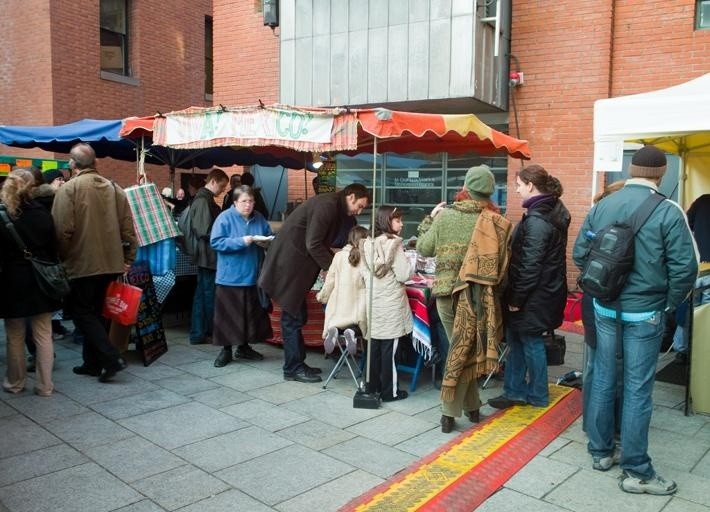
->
[465,166,495,202]
[630,146,666,177]
[43,168,64,184]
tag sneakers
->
[488,396,526,408]
[593,446,622,471]
[235,347,264,360]
[618,471,676,495]
[344,328,358,355]
[213,350,233,367]
[465,409,480,423]
[441,415,454,433]
[324,327,338,354]
[382,390,408,403]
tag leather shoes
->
[98,356,126,382]
[284,368,322,383]
[72,364,101,376]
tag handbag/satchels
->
[577,222,634,303]
[30,258,71,299]
[542,335,565,365]
[103,280,142,326]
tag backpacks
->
[178,195,206,255]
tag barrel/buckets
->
[545,332,566,365]
[564,289,583,322]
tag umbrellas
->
[0,115,324,198]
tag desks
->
[393,275,442,392]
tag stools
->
[322,334,363,392]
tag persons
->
[573,146,701,495]
[257,182,372,383]
[486,165,571,410]
[316,227,370,357]
[50,143,137,382]
[209,185,273,368]
[360,206,414,402]
[672,193,710,359]
[161,169,269,345]
[415,166,512,433]
[1,166,68,397]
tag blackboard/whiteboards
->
[127,260,168,366]
